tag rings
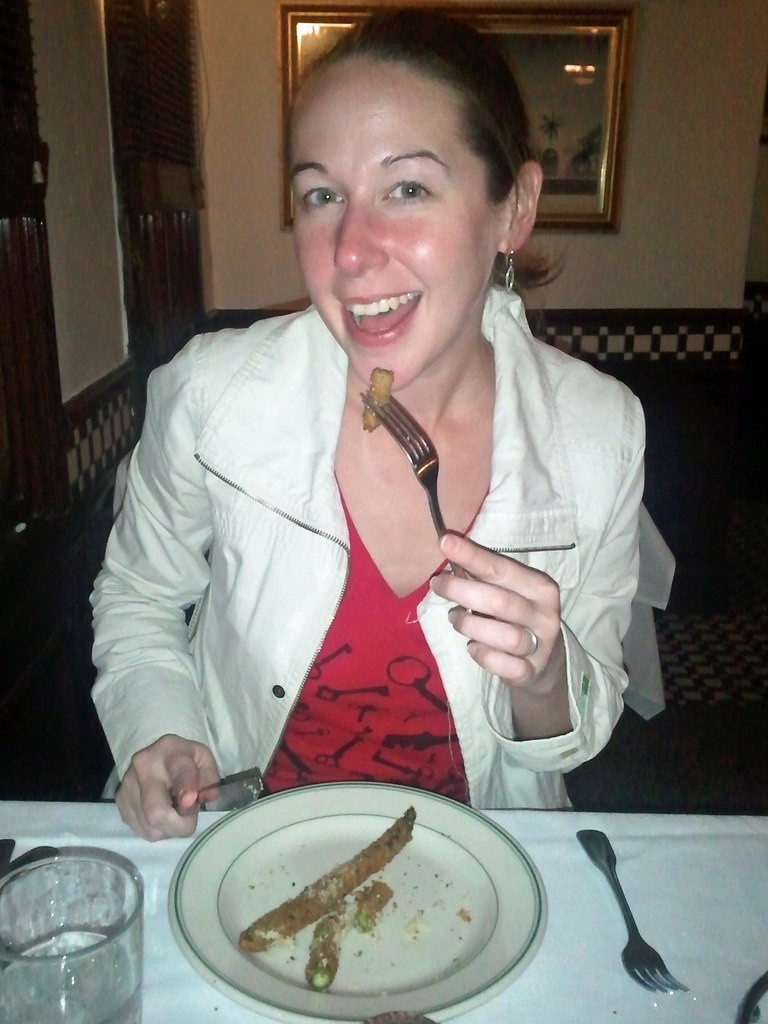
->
[523,628,539,657]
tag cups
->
[0,855,142,1023]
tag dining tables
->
[0,800,768,1024]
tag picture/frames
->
[276,4,637,236]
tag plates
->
[168,781,550,1024]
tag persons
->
[89,13,645,843]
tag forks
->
[577,829,690,995]
[360,389,467,582]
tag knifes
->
[171,778,263,813]
